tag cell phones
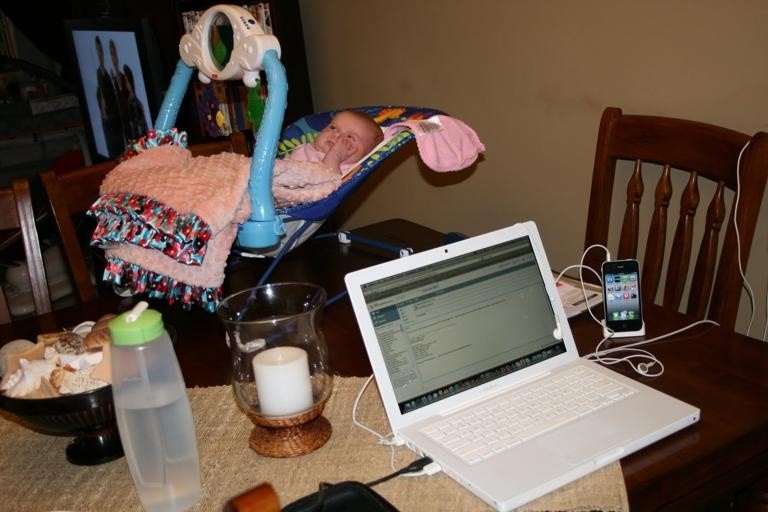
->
[601,258,642,333]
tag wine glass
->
[213,280,338,455]
[0,311,168,466]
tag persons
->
[123,63,148,143]
[94,34,125,160]
[280,110,387,179]
[108,38,133,146]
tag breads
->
[78,314,128,353]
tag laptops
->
[344,219,700,512]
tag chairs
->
[582,107,768,332]
[39,134,250,304]
[1,179,51,324]
[224,482,282,512]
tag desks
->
[0,216,768,512]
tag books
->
[180,2,276,139]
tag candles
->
[252,345,314,419]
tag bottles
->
[106,301,201,508]
